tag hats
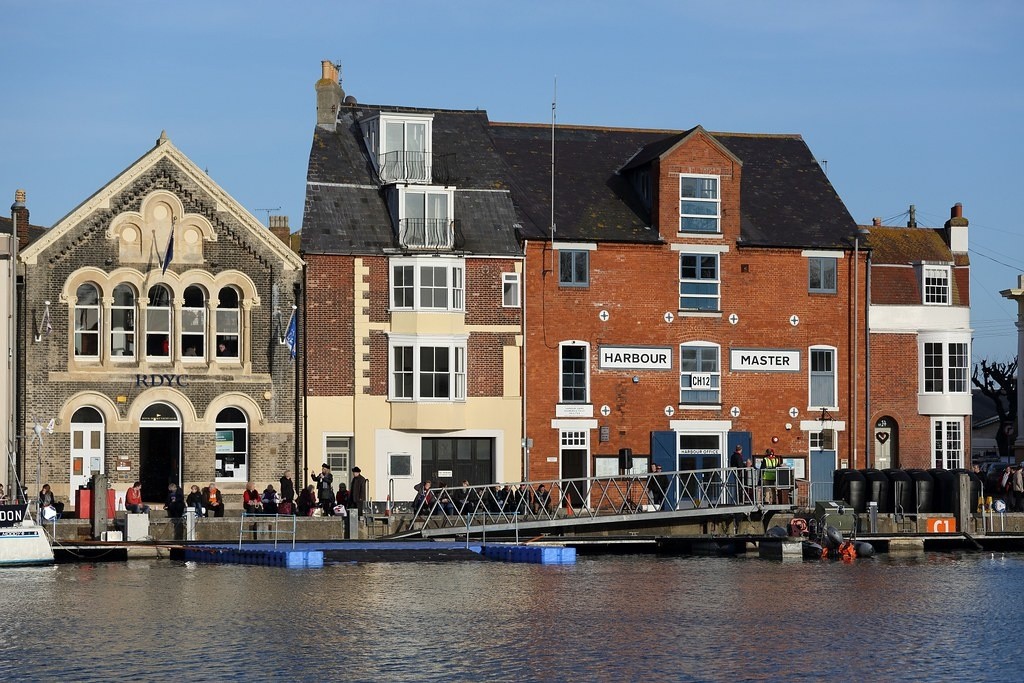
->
[735,445,742,451]
[352,467,361,472]
[766,448,774,455]
[322,463,330,469]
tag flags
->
[285,313,298,362]
[162,228,174,274]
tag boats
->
[0,434,57,566]
[760,517,875,562]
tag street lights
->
[853,227,871,473]
[8,200,28,500]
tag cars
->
[972,455,1022,485]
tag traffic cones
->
[385,495,393,516]
[566,494,573,515]
[117,498,124,511]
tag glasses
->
[657,468,662,469]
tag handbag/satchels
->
[43,505,56,520]
[278,500,291,514]
[309,506,324,517]
[333,504,347,516]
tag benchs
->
[116,509,246,519]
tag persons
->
[243,482,263,517]
[0,484,5,505]
[161,337,169,355]
[163,483,184,540]
[974,464,1024,512]
[260,484,280,517]
[125,481,150,514]
[646,462,670,511]
[411,478,551,516]
[730,444,798,505]
[185,345,197,356]
[280,470,294,516]
[295,463,367,516]
[186,484,205,518]
[39,484,53,511]
[201,483,224,517]
[219,343,229,356]
